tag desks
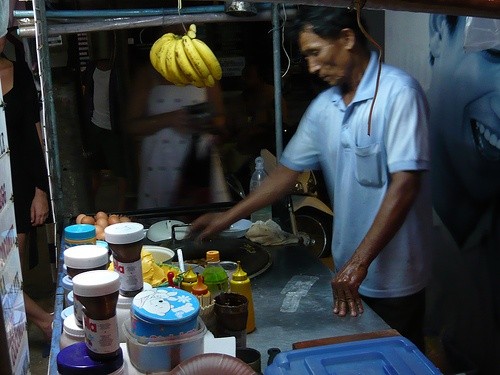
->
[47,217,398,375]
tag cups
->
[213,292,249,331]
[236,347,261,375]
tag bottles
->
[249,157,272,222]
[202,250,229,299]
[228,260,256,334]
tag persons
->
[423,13,500,356]
[84,60,127,213]
[24,290,55,339]
[186,7,431,354]
[121,25,230,211]
[225,56,287,173]
[0,36,49,267]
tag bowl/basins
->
[219,217,252,238]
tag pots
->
[158,237,274,280]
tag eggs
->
[76,212,132,241]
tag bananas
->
[150,25,222,88]
